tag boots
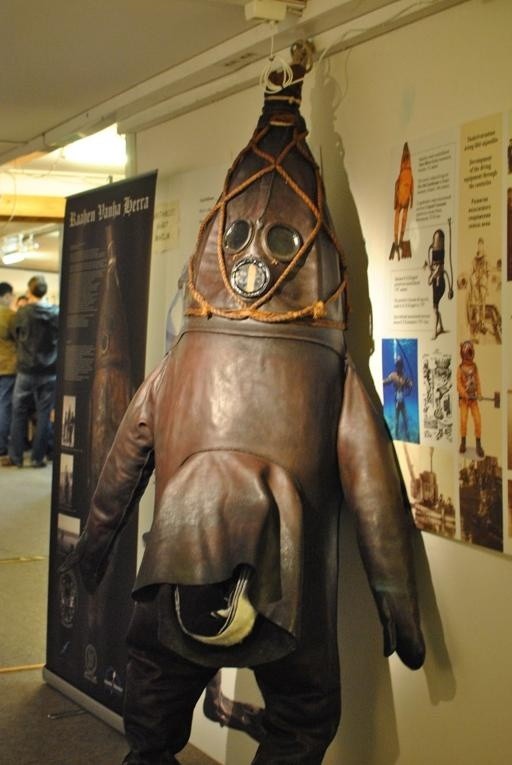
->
[459,436,467,453]
[476,437,485,457]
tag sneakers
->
[32,460,47,468]
[3,457,16,466]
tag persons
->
[383,356,415,441]
[0,275,62,468]
[456,339,489,458]
